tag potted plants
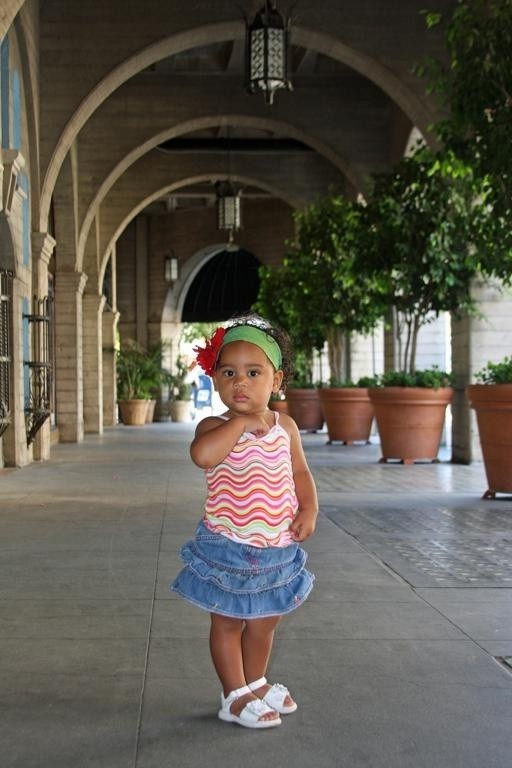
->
[367,157,501,471]
[394,2,509,501]
[105,339,192,426]
[250,259,326,433]
[285,167,375,445]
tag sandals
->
[218,677,298,729]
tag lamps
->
[216,125,244,248]
[163,248,179,291]
[236,0,299,109]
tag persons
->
[170,312,322,729]
